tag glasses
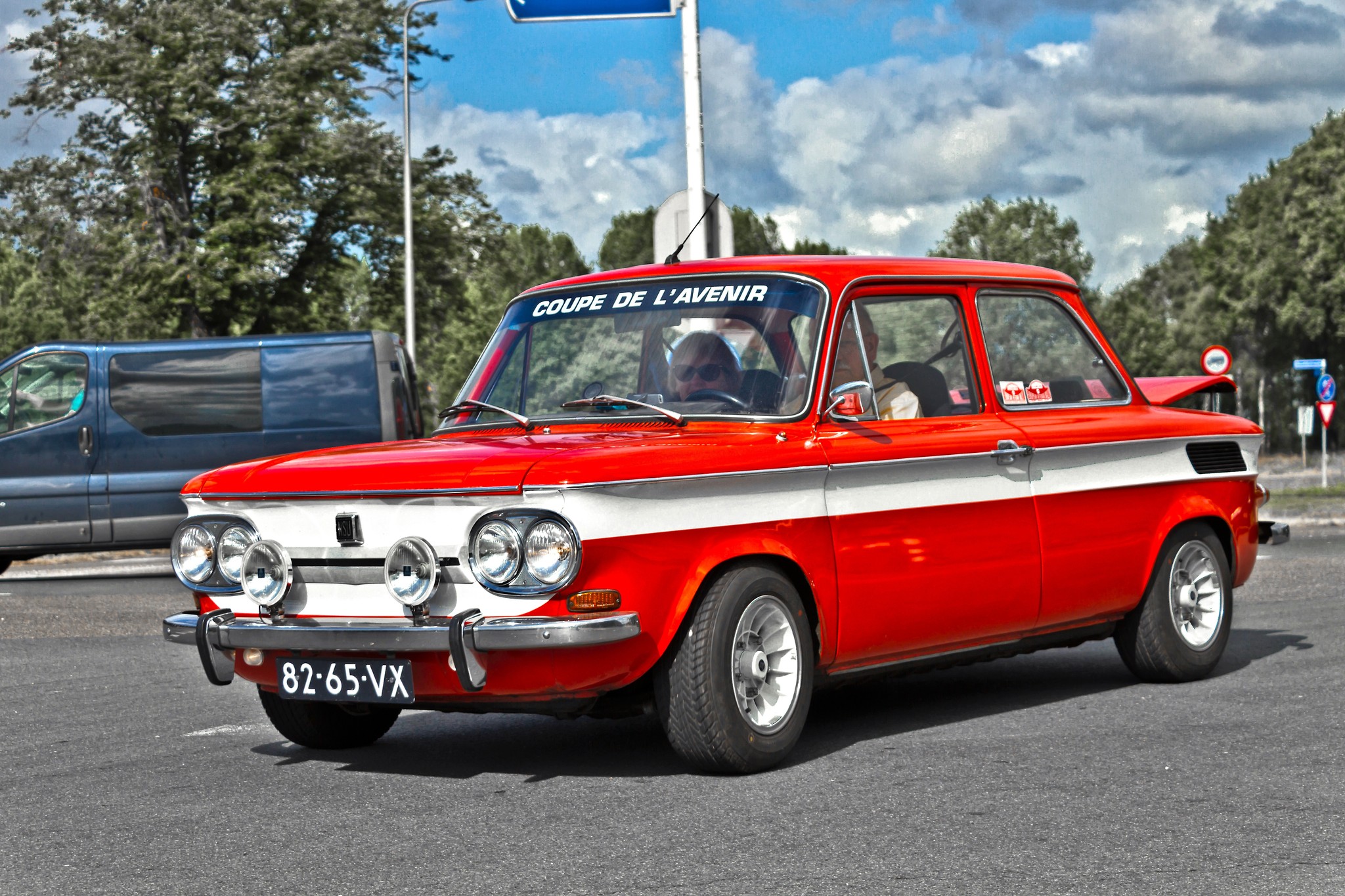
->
[672,364,731,382]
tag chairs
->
[881,361,951,417]
[739,370,781,412]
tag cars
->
[161,254,1290,774]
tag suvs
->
[0,329,423,573]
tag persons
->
[671,333,740,404]
[778,302,925,420]
[7,361,86,428]
